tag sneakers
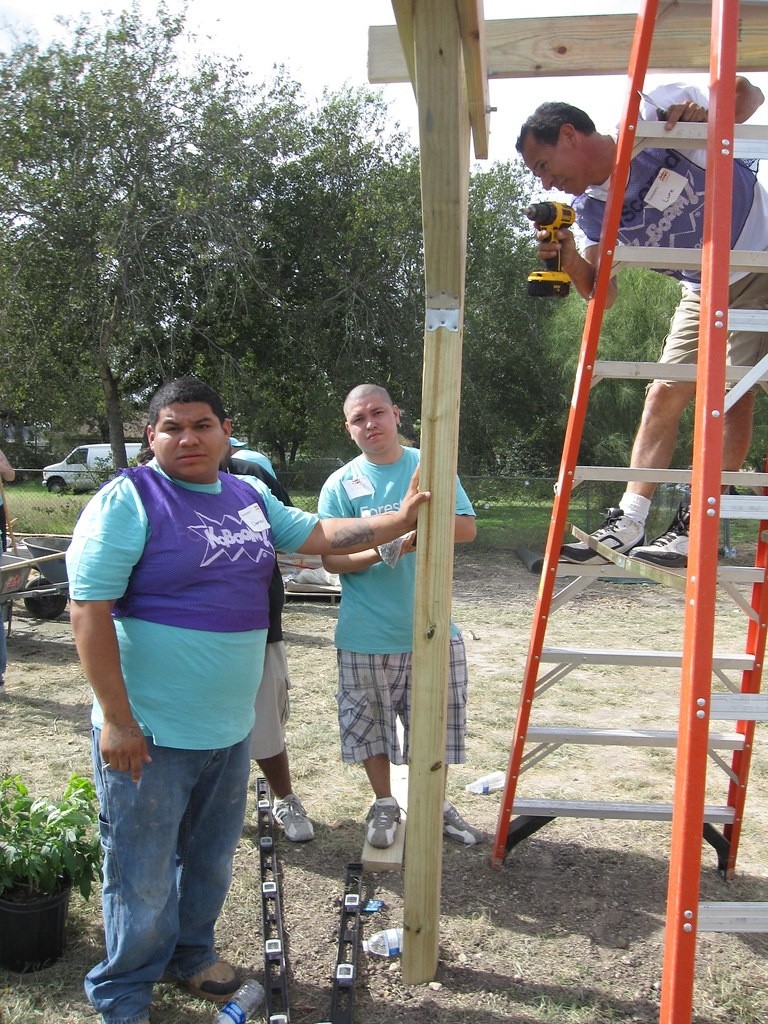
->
[365,796,407,848]
[443,799,484,844]
[272,792,315,842]
[629,498,691,567]
[560,507,646,565]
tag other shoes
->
[183,956,241,1003]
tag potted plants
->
[0,770,107,973]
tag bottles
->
[362,928,403,956]
[211,979,264,1024]
[466,771,506,793]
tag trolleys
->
[0,517,78,638]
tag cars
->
[663,460,760,496]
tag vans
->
[42,442,143,495]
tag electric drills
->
[519,200,576,298]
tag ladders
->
[495,0,768,1024]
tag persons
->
[514,75,768,568]
[0,449,15,684]
[317,384,484,849]
[64,377,433,1024]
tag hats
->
[229,438,247,447]
